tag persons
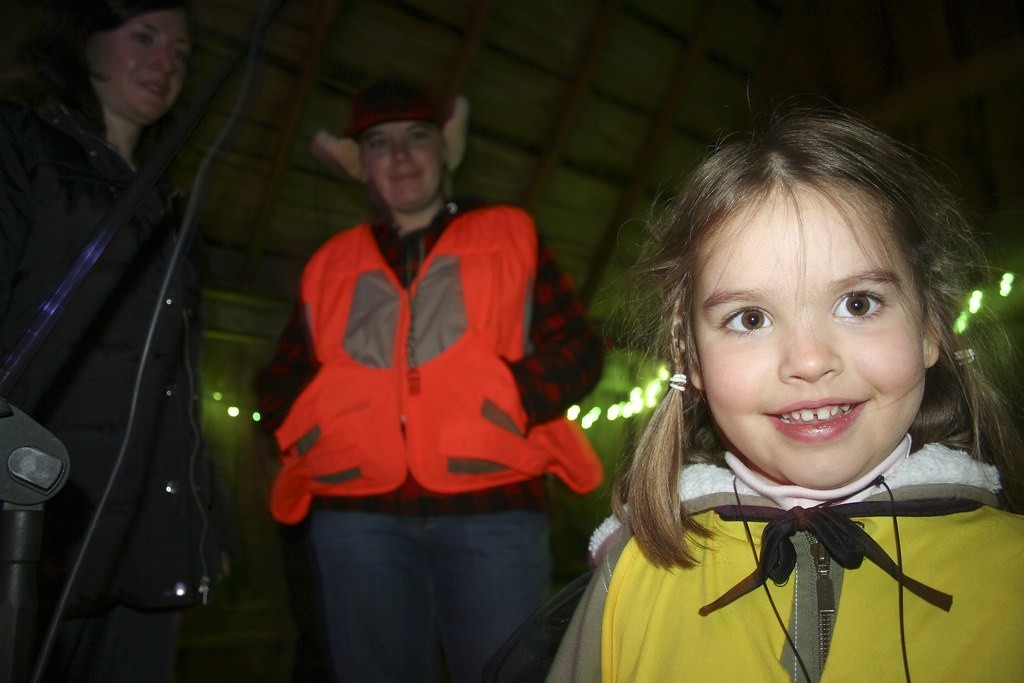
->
[543,109,1024,683]
[0,0,603,683]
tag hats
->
[344,79,452,137]
